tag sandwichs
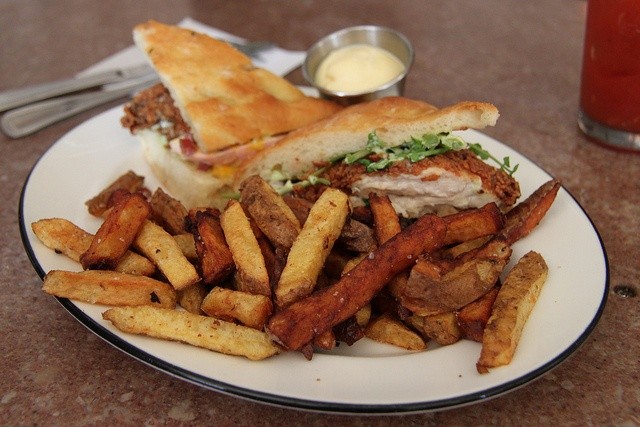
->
[122,19,344,184]
[210,96,521,220]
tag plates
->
[17,85,610,416]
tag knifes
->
[0,29,287,139]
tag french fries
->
[130,217,199,290]
[477,251,549,374]
[149,188,188,235]
[404,248,453,299]
[334,318,364,346]
[32,219,155,276]
[240,174,299,247]
[455,276,502,342]
[177,285,207,315]
[87,172,144,217]
[312,328,338,351]
[269,213,449,351]
[355,304,372,327]
[201,286,274,330]
[407,313,437,340]
[172,232,200,260]
[440,236,512,310]
[364,311,427,350]
[102,307,280,360]
[369,191,409,298]
[40,270,177,310]
[275,188,350,309]
[423,310,463,346]
[185,212,234,285]
[292,183,375,225]
[449,178,561,259]
[79,193,152,270]
[219,201,271,296]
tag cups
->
[300,25,415,107]
[577,1,640,156]
